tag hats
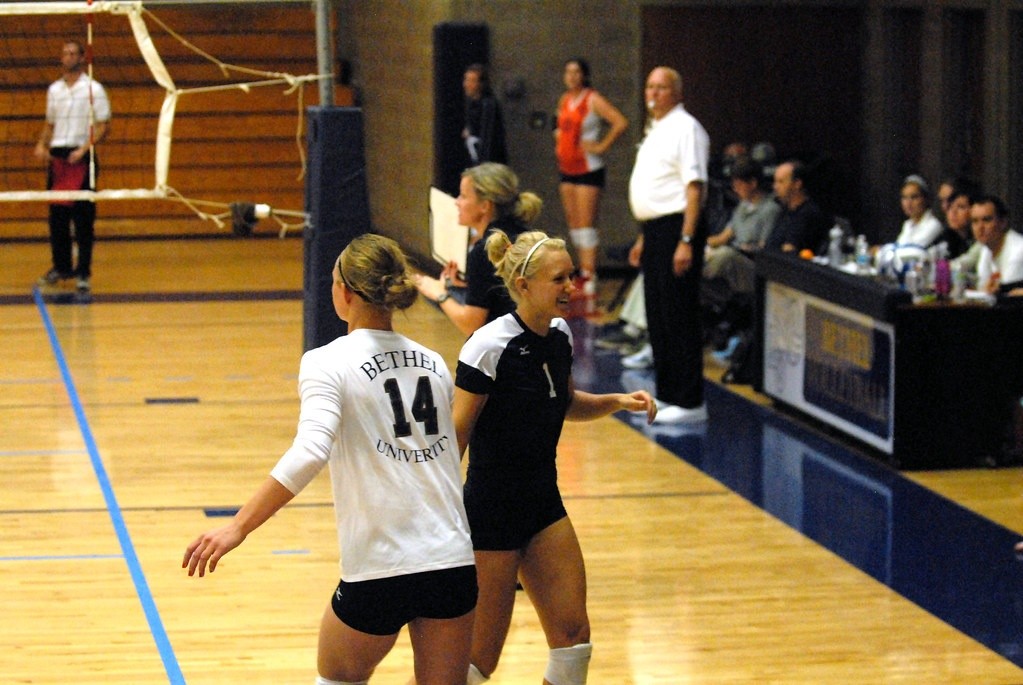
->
[724,144,748,160]
[750,142,775,160]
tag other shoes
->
[645,423,707,438]
[710,335,738,360]
[629,395,667,415]
[38,269,64,286]
[629,417,649,427]
[567,276,596,300]
[622,371,657,395]
[651,402,708,424]
[594,328,637,351]
[620,340,655,368]
[76,276,91,291]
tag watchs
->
[679,234,693,245]
[437,293,451,304]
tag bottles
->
[824,223,954,300]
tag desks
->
[739,246,1023,471]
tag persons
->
[459,63,508,165]
[592,144,834,385]
[551,55,629,297]
[181,232,479,685]
[405,163,544,591]
[35,39,112,292]
[449,230,658,685]
[863,175,1023,302]
[629,67,710,426]
